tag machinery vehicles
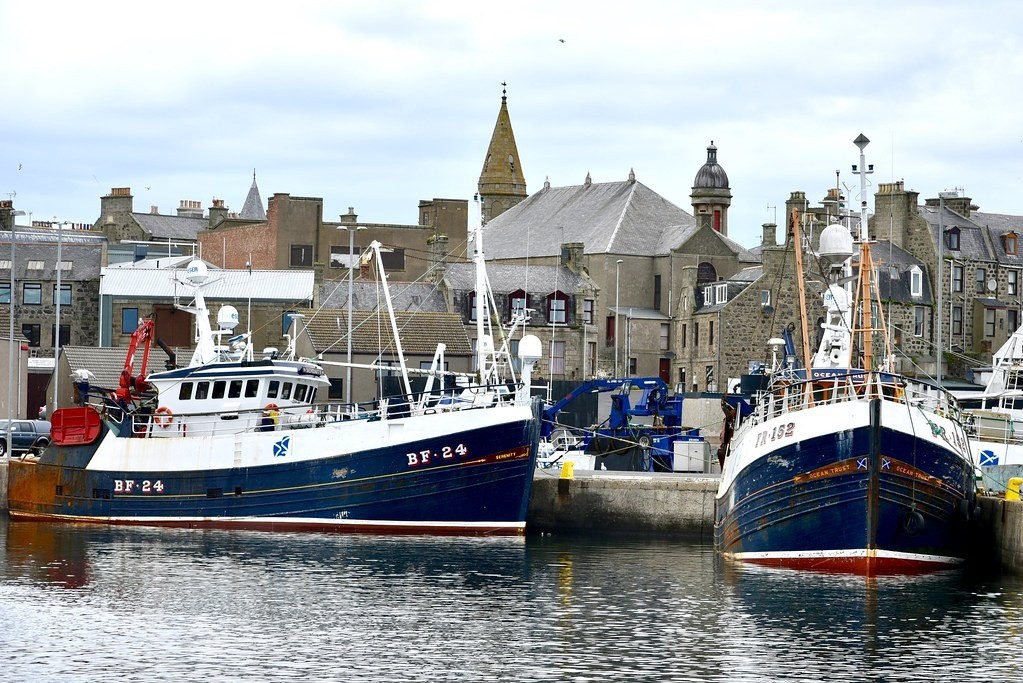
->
[542,377,682,443]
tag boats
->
[5,189,542,546]
[713,133,977,575]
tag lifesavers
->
[156,408,174,428]
[263,404,279,414]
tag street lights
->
[7,209,26,421]
[615,259,623,379]
[49,219,76,412]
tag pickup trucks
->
[0,420,53,458]
